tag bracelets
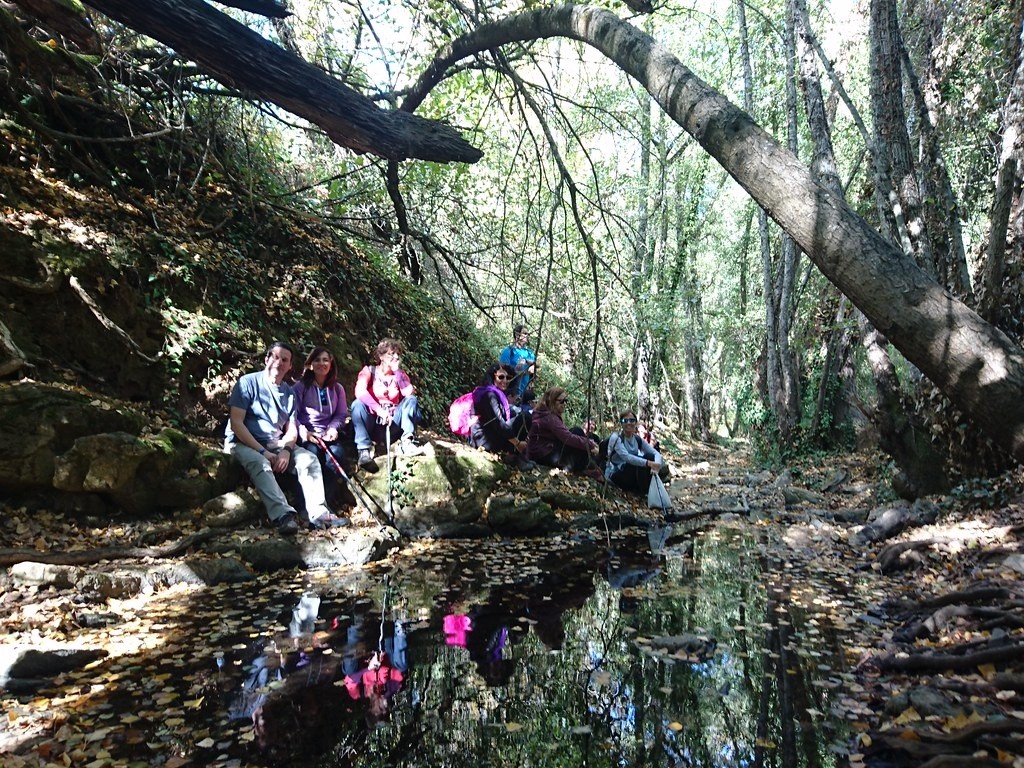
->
[517,441,521,445]
[283,446,292,452]
[258,447,266,454]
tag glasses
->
[319,388,328,406]
[620,418,636,423]
[555,398,568,404]
[384,381,389,396]
[494,373,513,380]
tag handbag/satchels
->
[648,471,673,508]
[647,527,672,558]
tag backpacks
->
[443,613,472,648]
[448,387,501,436]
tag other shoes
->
[279,511,298,535]
[397,440,424,456]
[309,510,350,532]
[357,448,374,468]
[501,450,536,471]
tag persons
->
[224,342,351,535]
[500,325,536,396]
[291,347,348,476]
[506,386,670,490]
[470,363,537,471]
[350,338,418,467]
